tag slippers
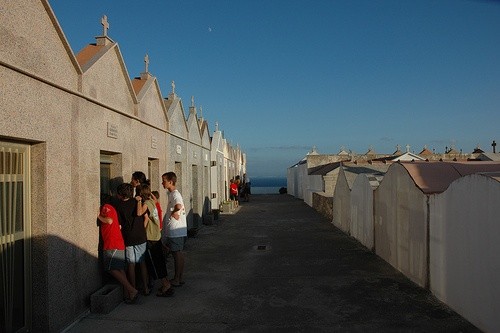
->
[122,296,135,304]
[143,289,152,296]
[173,281,185,287]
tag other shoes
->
[155,287,175,297]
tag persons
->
[230,174,251,203]
[162,172,187,287]
[110,171,176,297]
[97,193,138,304]
[113,184,152,304]
